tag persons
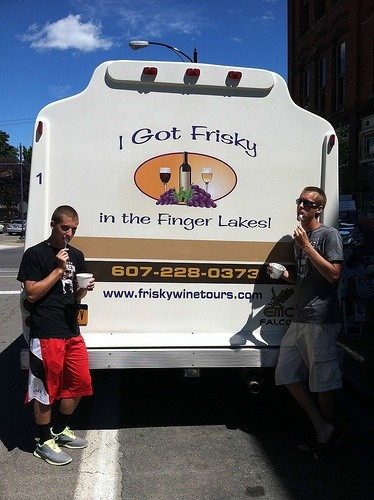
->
[16,206,94,466]
[267,186,344,463]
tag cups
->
[270,263,286,279]
[76,272,93,288]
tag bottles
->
[179,151,192,202]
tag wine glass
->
[201,167,213,192]
[159,167,171,193]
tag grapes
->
[155,189,178,205]
[186,185,217,208]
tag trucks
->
[19,59,342,395]
[338,194,358,245]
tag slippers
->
[311,423,346,463]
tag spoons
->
[64,238,68,249]
[299,213,302,228]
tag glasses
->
[296,199,320,206]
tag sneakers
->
[50,426,87,449]
[34,437,72,466]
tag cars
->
[0,222,5,234]
[6,219,26,235]
